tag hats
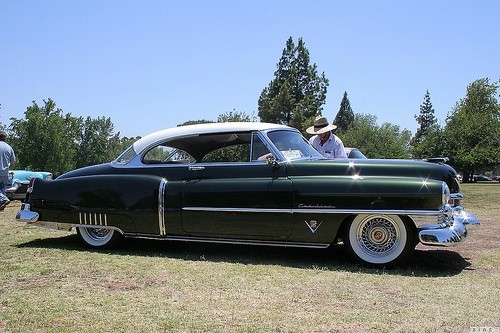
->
[306,116,338,134]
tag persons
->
[306,116,349,159]
[0,131,17,211]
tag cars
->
[3,170,54,203]
[16,123,481,267]
[456,172,500,183]
